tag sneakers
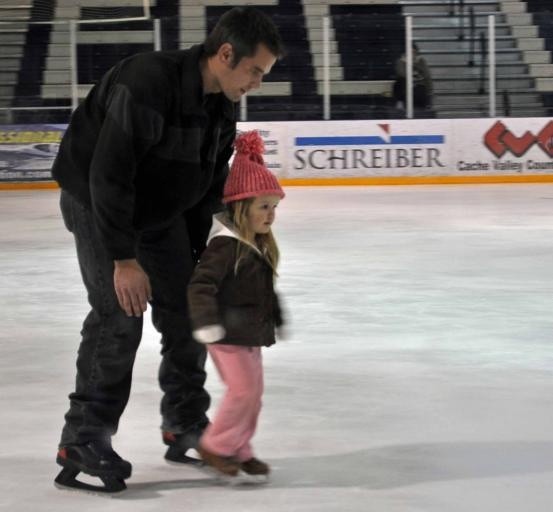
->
[162,422,210,447]
[241,458,267,475]
[197,447,239,475]
[57,439,132,479]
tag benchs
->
[0,0,551,118]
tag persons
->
[183,127,291,482]
[47,6,291,480]
[394,82,431,117]
[393,40,436,108]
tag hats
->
[220,129,285,203]
